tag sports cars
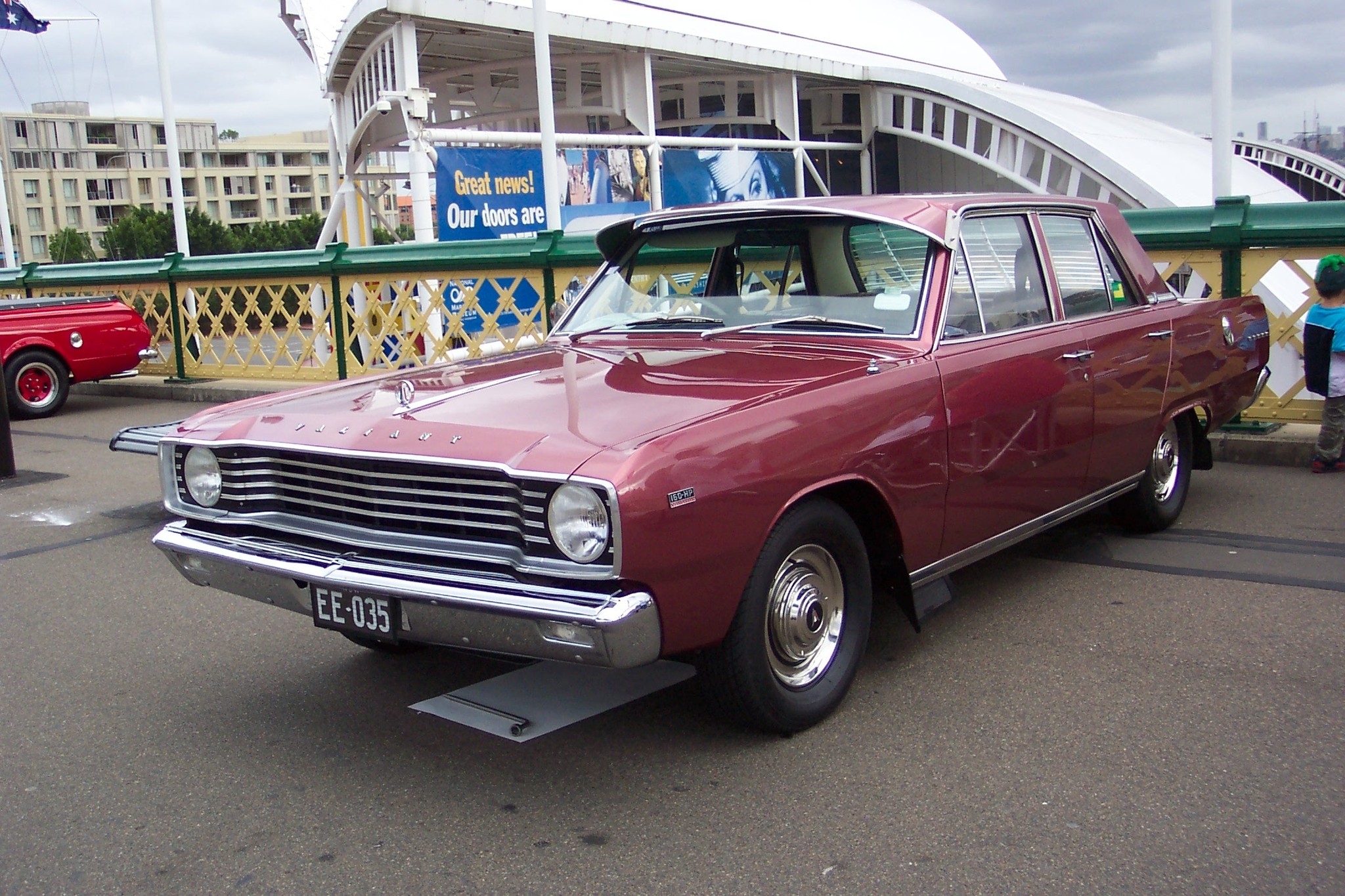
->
[1,291,161,422]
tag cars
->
[149,195,1279,740]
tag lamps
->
[374,178,390,198]
[402,179,410,189]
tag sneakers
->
[1312,455,1345,473]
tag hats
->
[698,151,759,192]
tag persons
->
[698,150,784,204]
[556,147,651,205]
[1302,254,1345,472]
